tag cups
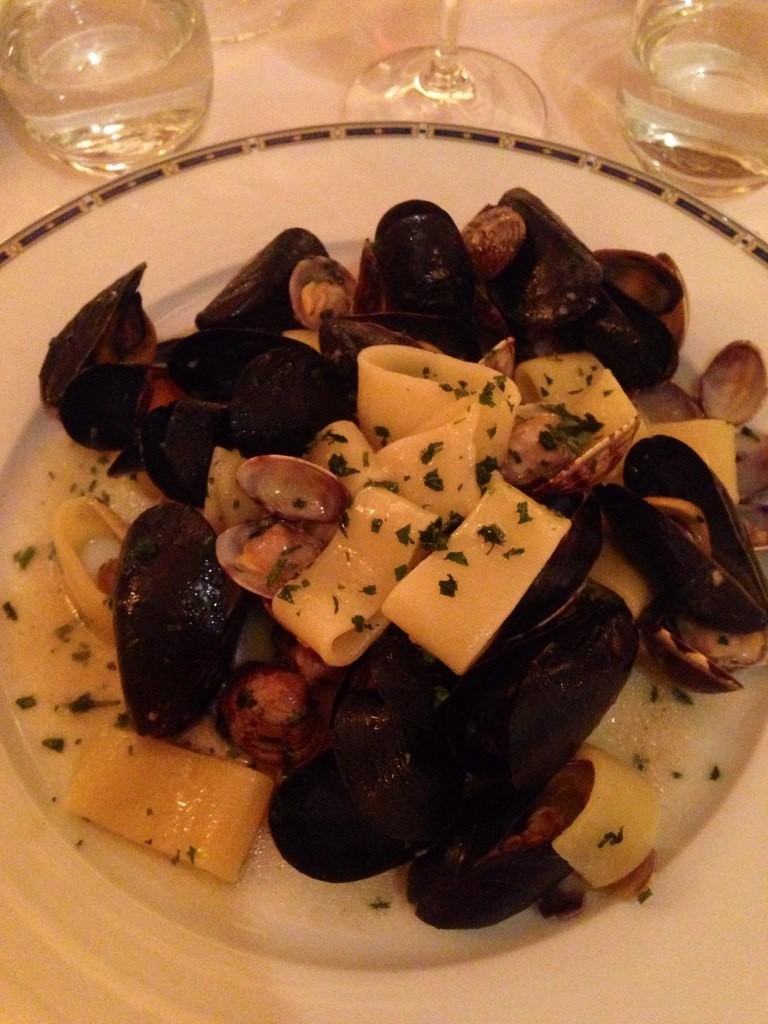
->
[617,0,768,197]
[0,0,215,180]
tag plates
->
[0,122,768,1024]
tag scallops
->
[38,187,767,930]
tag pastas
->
[50,344,738,881]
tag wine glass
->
[345,0,548,143]
